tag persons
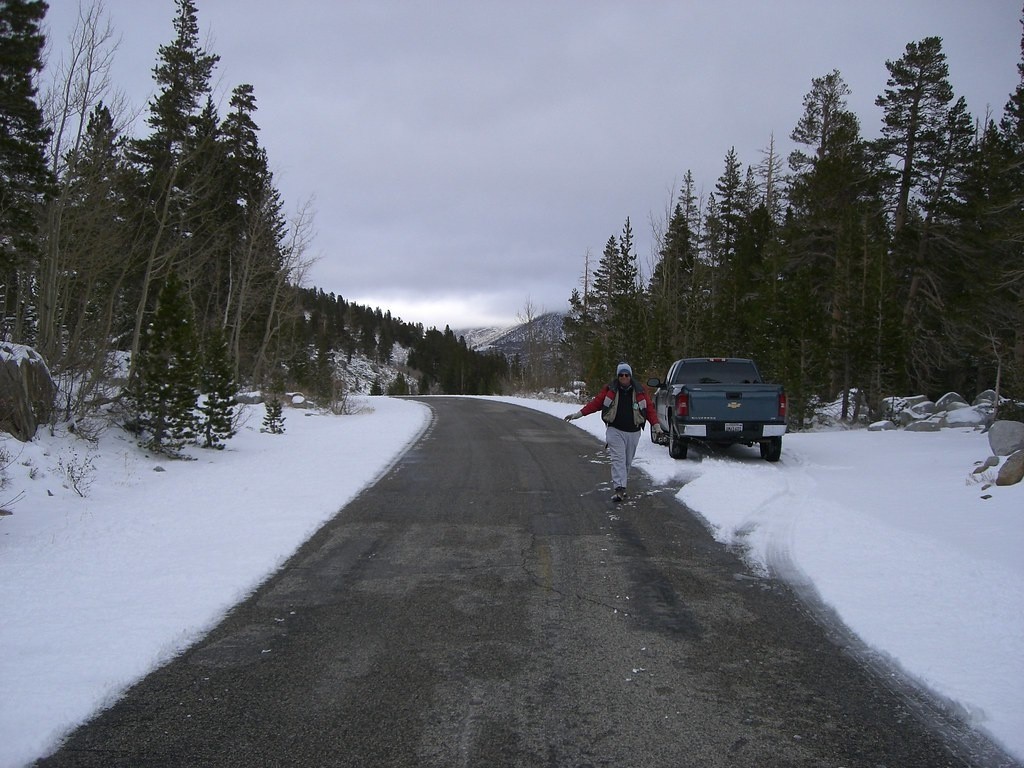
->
[564,362,664,501]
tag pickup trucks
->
[647,357,791,463]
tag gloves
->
[654,423,663,434]
[564,410,583,423]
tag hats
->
[617,362,632,378]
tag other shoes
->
[623,491,627,496]
[611,487,624,501]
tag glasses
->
[619,374,630,377]
